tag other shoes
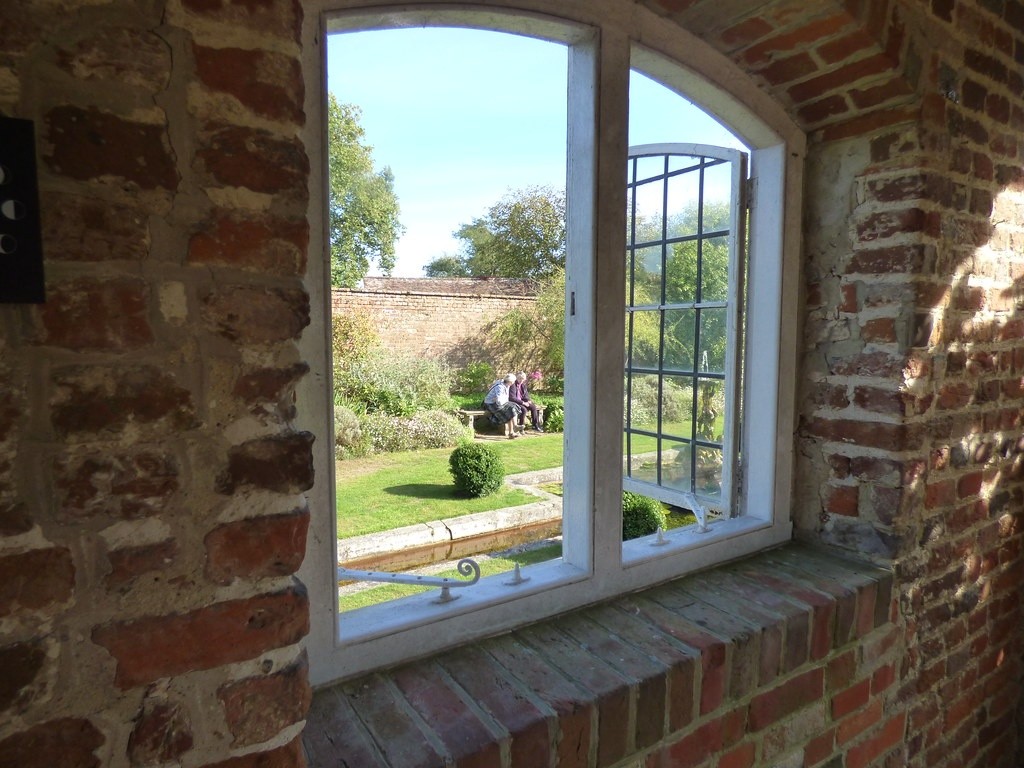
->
[508,432,519,439]
[520,429,526,435]
[532,423,544,433]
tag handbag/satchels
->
[480,399,489,410]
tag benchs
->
[457,405,548,436]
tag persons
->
[508,373,541,432]
[483,375,526,439]
